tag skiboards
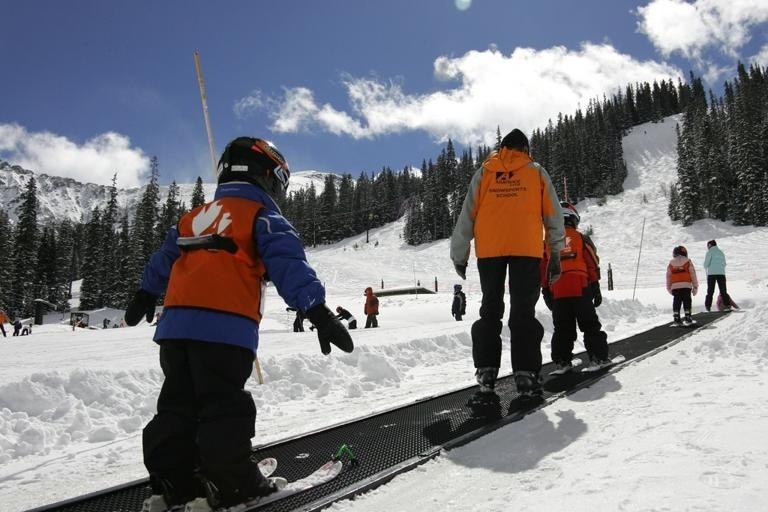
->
[465,392,546,417]
[704,308,733,312]
[669,320,697,327]
[141,457,342,512]
[548,354,626,376]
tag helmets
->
[672,246,686,257]
[216,136,291,206]
[559,201,580,228]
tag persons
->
[702,239,731,312]
[666,245,700,326]
[18,322,33,335]
[286,307,305,332]
[119,318,125,327]
[450,284,467,322]
[335,305,358,329]
[0,307,13,337]
[156,312,162,323]
[363,286,380,328]
[123,132,356,511]
[112,324,119,328]
[9,318,22,336]
[103,318,108,329]
[535,199,615,369]
[448,126,568,400]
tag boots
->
[673,313,682,323]
[684,313,691,322]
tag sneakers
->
[588,355,611,365]
[199,468,278,509]
[146,472,208,504]
[556,359,573,368]
[475,367,497,388]
[515,368,542,389]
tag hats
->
[501,128,529,155]
[708,240,715,246]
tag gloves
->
[691,287,696,296]
[667,288,672,294]
[305,301,353,355]
[546,252,561,285]
[454,261,468,280]
[541,287,554,310]
[592,281,601,307]
[124,289,157,327]
